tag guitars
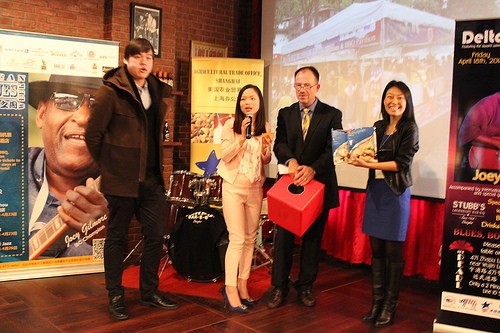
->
[28,175,102,260]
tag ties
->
[302,107,311,143]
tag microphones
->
[245,112,253,139]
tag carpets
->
[101,242,274,303]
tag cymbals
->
[159,141,184,146]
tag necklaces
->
[379,130,394,150]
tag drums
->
[207,169,226,207]
[165,168,204,208]
[166,205,231,284]
[254,214,276,246]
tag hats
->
[27,74,104,110]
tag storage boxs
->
[267,173,325,238]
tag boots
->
[361,259,388,322]
[373,259,406,327]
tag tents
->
[281,0,453,73]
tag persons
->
[347,80,419,326]
[137,13,157,49]
[269,66,344,308]
[459,91,500,167]
[322,54,453,93]
[218,85,274,313]
[85,39,180,320]
[27,74,107,258]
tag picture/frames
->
[130,2,162,57]
[187,40,229,103]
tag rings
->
[305,178,307,180]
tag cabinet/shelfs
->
[162,90,184,147]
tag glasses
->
[293,83,318,90]
[48,89,96,112]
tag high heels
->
[219,285,250,313]
[239,297,256,307]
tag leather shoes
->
[297,288,316,306]
[266,286,286,309]
[108,294,129,320]
[139,290,180,310]
